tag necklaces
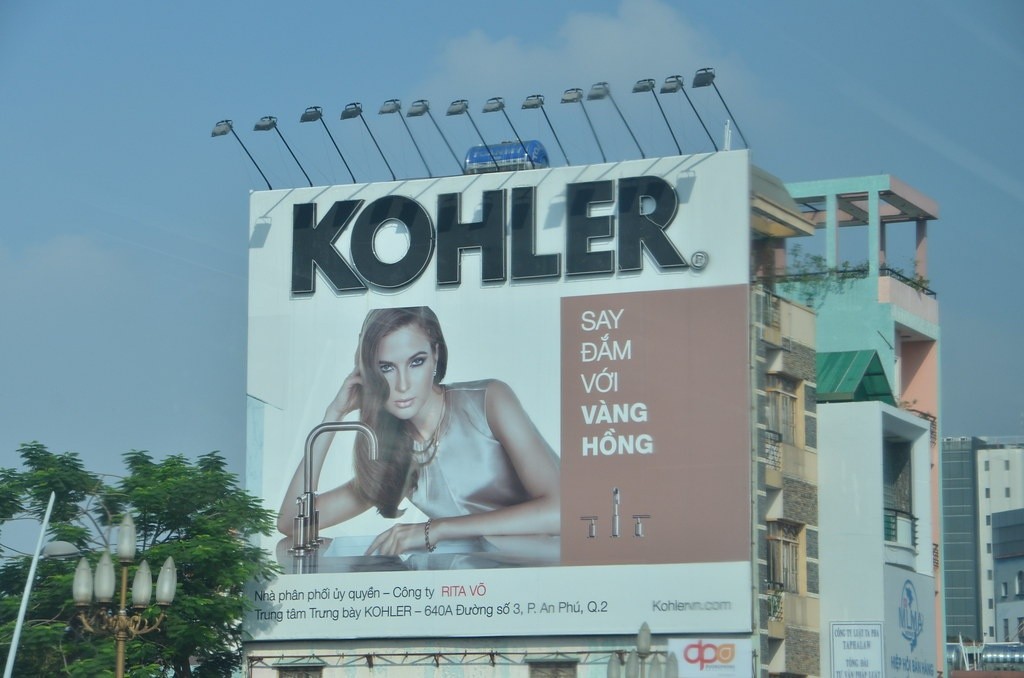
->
[413,386,446,467]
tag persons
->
[278,307,561,556]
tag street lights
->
[605,620,681,678]
[71,511,177,678]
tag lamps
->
[212,66,748,190]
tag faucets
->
[286,421,379,556]
[609,487,621,539]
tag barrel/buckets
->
[979,644,1024,671]
[463,140,549,174]
[947,644,965,671]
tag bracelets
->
[425,518,435,552]
